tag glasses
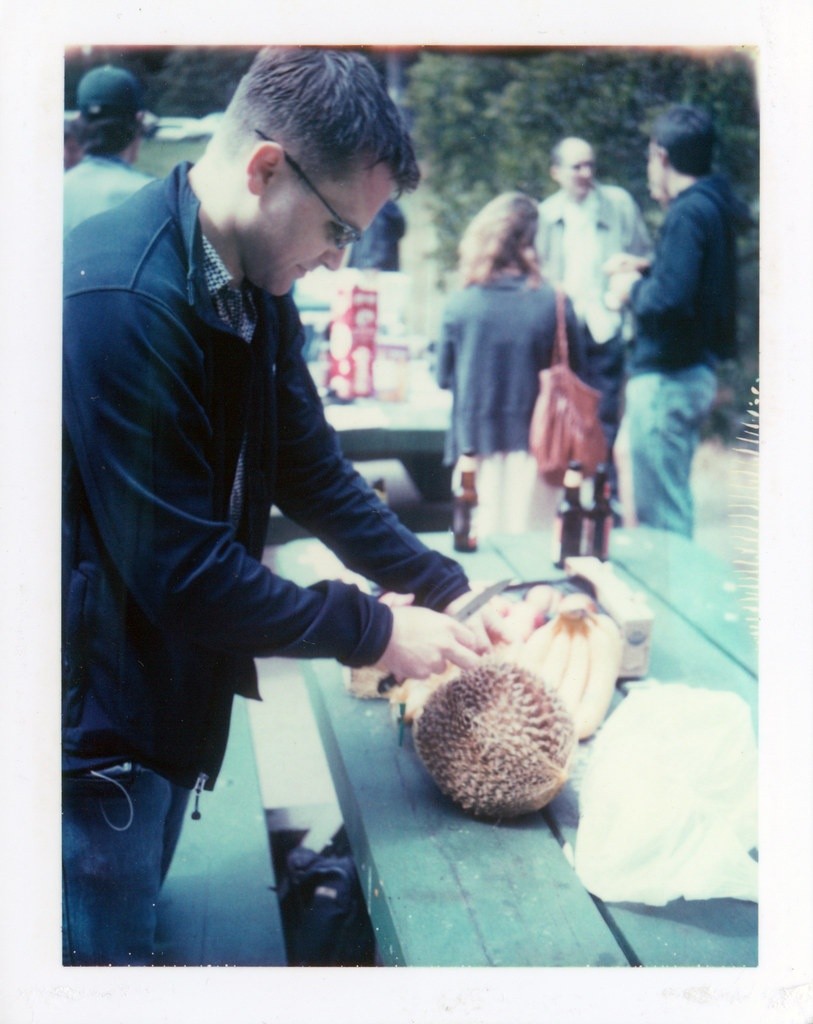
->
[254,127,361,251]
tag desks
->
[274,527,759,967]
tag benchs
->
[152,692,288,967]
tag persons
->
[61,45,524,968]
[61,65,727,536]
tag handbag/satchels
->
[529,291,609,487]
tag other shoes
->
[592,499,609,516]
[562,499,582,516]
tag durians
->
[412,658,575,816]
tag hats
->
[76,64,143,114]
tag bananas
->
[503,603,622,739]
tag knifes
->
[376,578,514,696]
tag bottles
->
[554,460,583,569]
[452,446,481,552]
[583,463,616,560]
